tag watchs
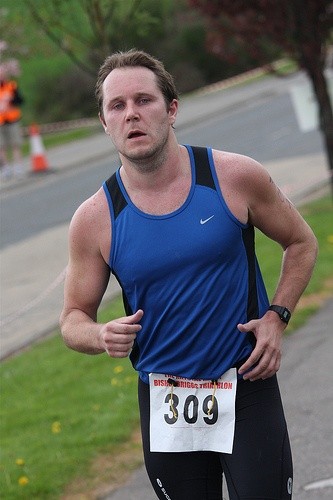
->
[268,305,292,326]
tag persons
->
[0,63,28,181]
[59,52,318,500]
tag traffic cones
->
[27,125,54,176]
